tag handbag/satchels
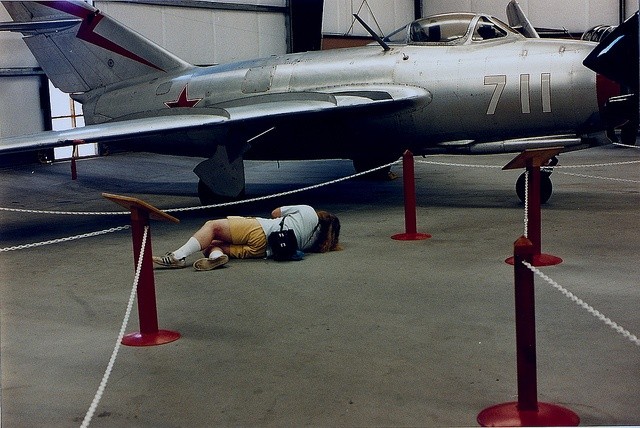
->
[267,215,320,262]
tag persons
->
[152,204,343,272]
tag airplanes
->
[0,2,640,208]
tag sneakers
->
[193,253,228,271]
[152,252,187,267]
